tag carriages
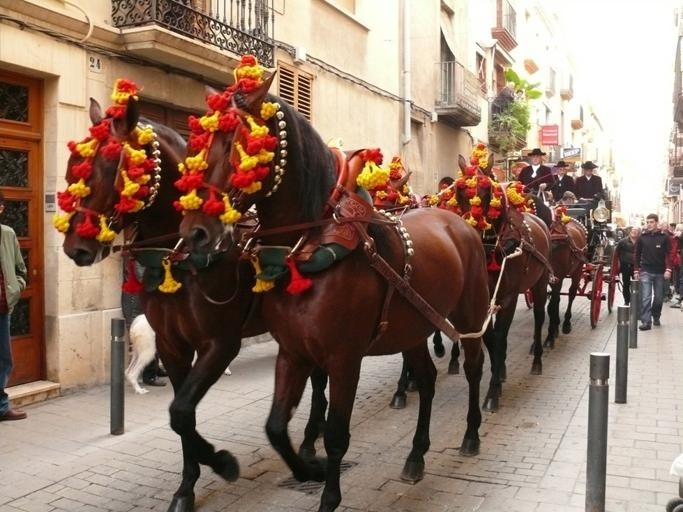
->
[61,70,621,512]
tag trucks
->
[599,222,633,274]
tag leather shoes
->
[157,368,169,377]
[147,376,167,387]
[653,317,660,326]
[638,322,651,330]
[1,409,27,420]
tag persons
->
[0,192,27,420]
[552,161,576,201]
[518,149,556,200]
[615,214,683,330]
[574,161,602,208]
[491,81,516,121]
[516,89,524,97]
[122,259,168,386]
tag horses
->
[64,96,327,512]
[456,155,552,412]
[524,186,588,355]
[368,171,459,409]
[177,68,490,512]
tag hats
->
[528,148,546,156]
[554,160,569,169]
[581,161,598,169]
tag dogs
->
[123,314,231,394]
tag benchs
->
[563,208,588,228]
